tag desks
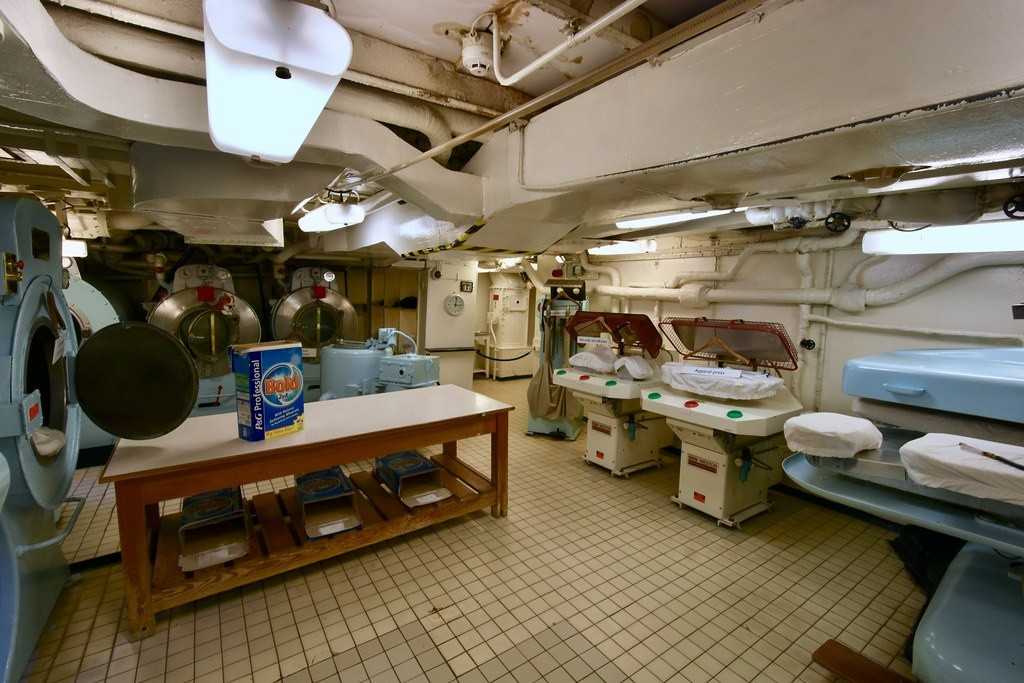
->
[99,383,515,643]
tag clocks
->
[444,292,465,315]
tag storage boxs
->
[294,467,363,538]
[229,340,306,441]
[376,450,453,508]
[176,487,250,573]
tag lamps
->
[588,239,657,255]
[862,220,1024,255]
[204,0,353,167]
[297,203,364,232]
